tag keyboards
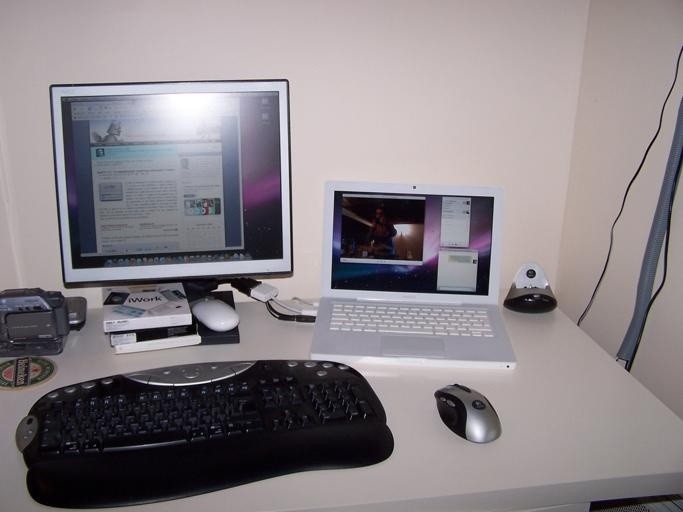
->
[15,358,394,508]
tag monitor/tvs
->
[49,78,294,343]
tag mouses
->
[433,383,501,443]
[193,296,239,332]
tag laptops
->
[307,180,517,374]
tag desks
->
[0,299,683,512]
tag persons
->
[365,207,396,257]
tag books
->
[100,284,191,334]
[108,308,202,354]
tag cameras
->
[0,288,71,357]
